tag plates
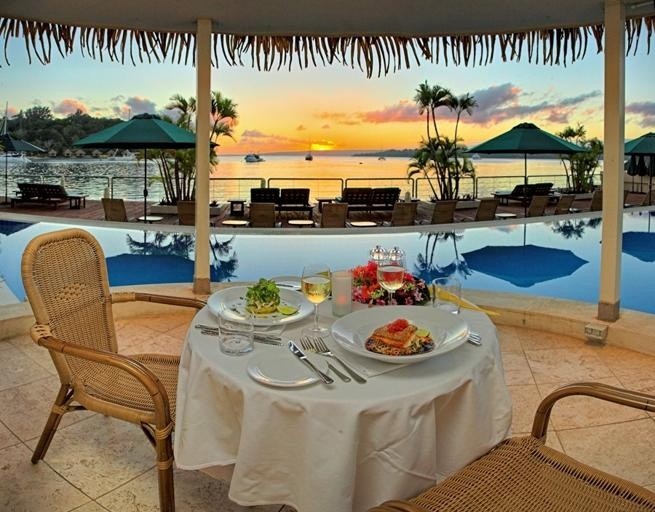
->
[271,275,302,290]
[206,285,315,325]
[249,352,328,386]
[333,303,470,365]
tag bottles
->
[369,245,405,261]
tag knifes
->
[287,340,333,384]
[194,323,282,346]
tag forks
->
[299,335,367,387]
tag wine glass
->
[301,264,332,339]
[375,257,405,305]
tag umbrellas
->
[72,112,220,225]
[461,122,590,218]
[461,217,589,288]
[0,220,38,237]
[0,134,47,202]
[104,231,213,286]
[600,211,655,262]
[624,131,655,206]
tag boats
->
[304,153,315,161]
[243,152,264,162]
[4,151,22,157]
[377,155,386,160]
[471,152,482,161]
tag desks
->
[227,201,246,216]
[174,286,513,512]
[67,194,89,209]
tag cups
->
[331,272,353,316]
[432,277,463,317]
[219,306,255,354]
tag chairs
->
[177,201,215,227]
[367,382,655,512]
[17,182,69,209]
[22,228,207,512]
[101,198,140,222]
